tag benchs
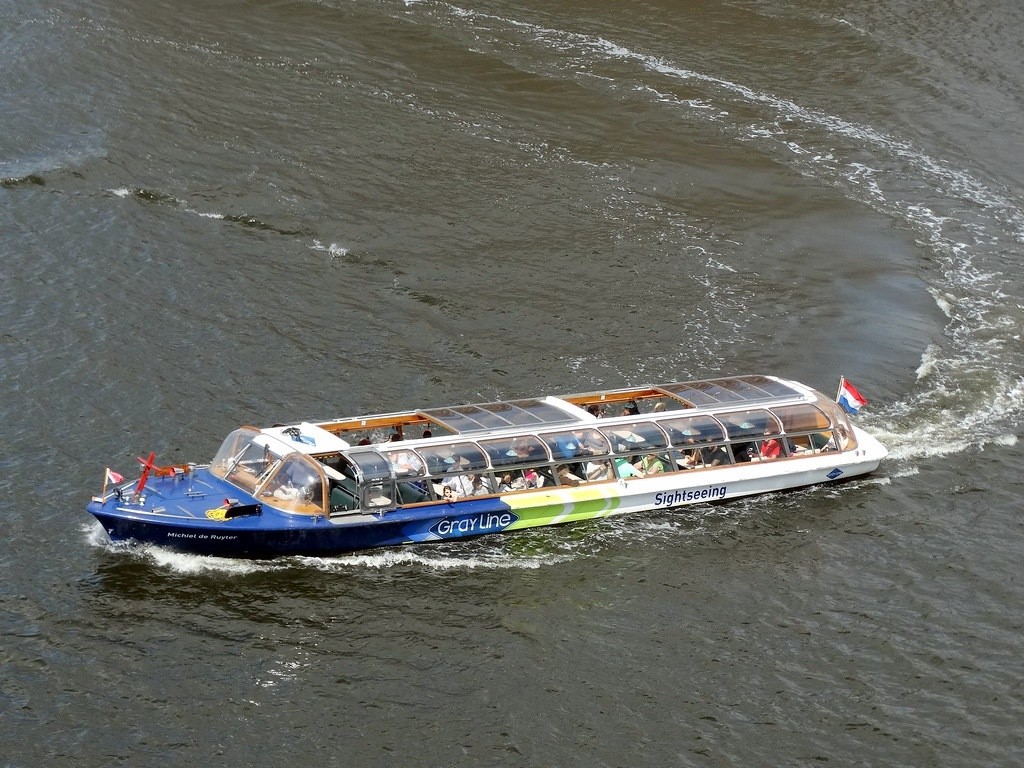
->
[397,482,431,504]
[425,452,443,483]
[635,448,673,472]
[468,444,503,477]
[518,462,556,487]
[330,485,359,509]
[339,473,359,494]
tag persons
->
[358,430,489,500]
[683,421,849,469]
[501,401,664,493]
[283,457,323,493]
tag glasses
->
[649,454,655,456]
[454,460,459,462]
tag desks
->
[720,446,767,462]
[613,430,647,445]
[668,422,687,432]
[664,454,710,469]
[795,445,807,453]
[432,483,459,497]
[729,415,744,426]
[371,495,391,505]
[548,469,584,482]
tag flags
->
[108,471,123,484]
[838,379,867,414]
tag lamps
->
[566,442,576,450]
[444,449,455,464]
[625,434,636,442]
[681,420,701,436]
[505,448,517,457]
[738,420,756,429]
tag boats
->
[87,375,887,562]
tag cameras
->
[603,459,610,467]
